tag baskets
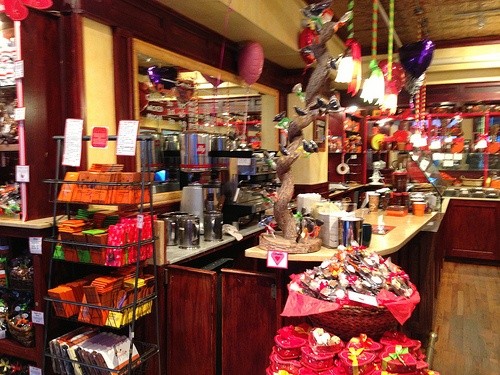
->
[286,272,420,342]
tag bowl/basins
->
[460,175,485,187]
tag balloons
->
[379,40,435,102]
[299,28,318,66]
[236,41,264,85]
[137,65,223,112]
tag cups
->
[338,216,364,247]
[176,214,200,250]
[362,223,372,248]
[203,211,223,241]
[412,202,426,216]
[369,193,379,212]
[162,211,187,246]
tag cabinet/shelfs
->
[0,212,71,375]
[0,2,75,223]
[328,112,392,184]
[41,133,161,375]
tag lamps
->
[331,0,487,166]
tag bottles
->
[394,172,407,192]
[381,188,409,212]
[486,176,491,188]
